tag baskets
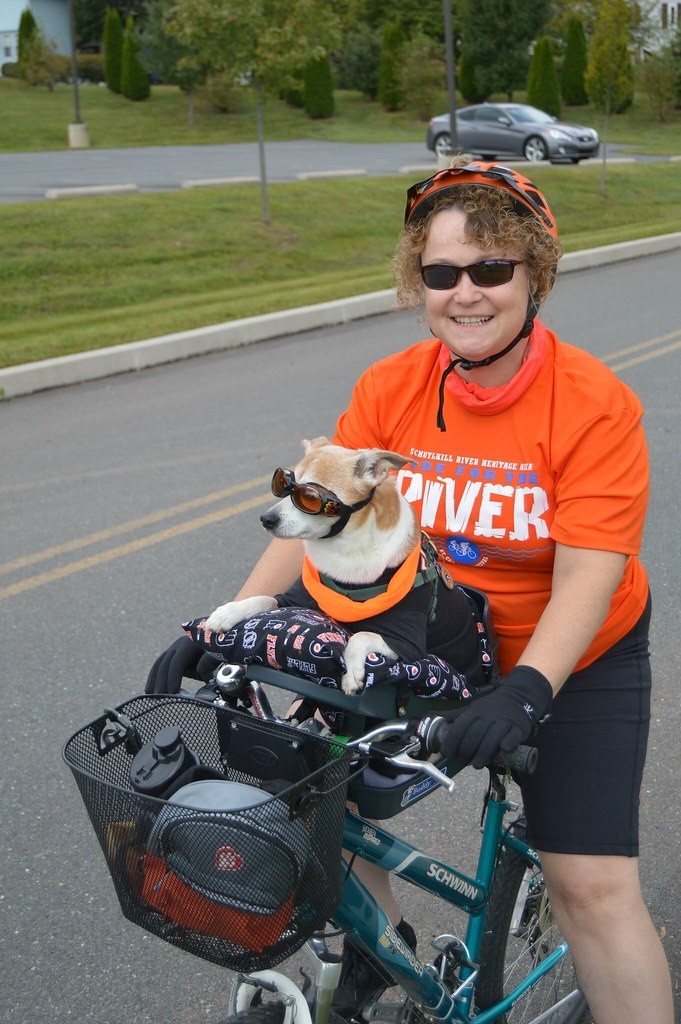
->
[61,693,353,973]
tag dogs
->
[204,435,492,695]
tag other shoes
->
[331,916,417,1021]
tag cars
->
[426,101,603,166]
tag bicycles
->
[64,612,595,1022]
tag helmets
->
[404,161,558,290]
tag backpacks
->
[141,780,311,952]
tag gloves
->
[144,636,219,695]
[430,664,554,770]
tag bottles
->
[131,725,203,801]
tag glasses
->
[420,259,523,290]
[271,467,377,517]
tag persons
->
[231,159,675,1024]
